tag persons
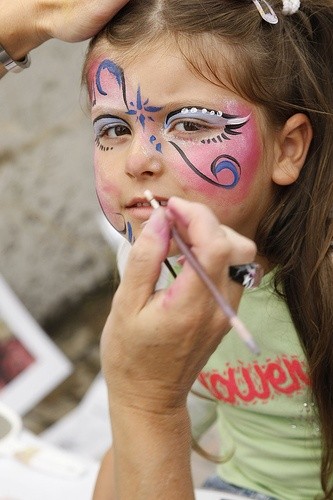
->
[80,0,333,500]
[0,0,258,500]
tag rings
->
[229,263,262,289]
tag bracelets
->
[0,39,31,73]
